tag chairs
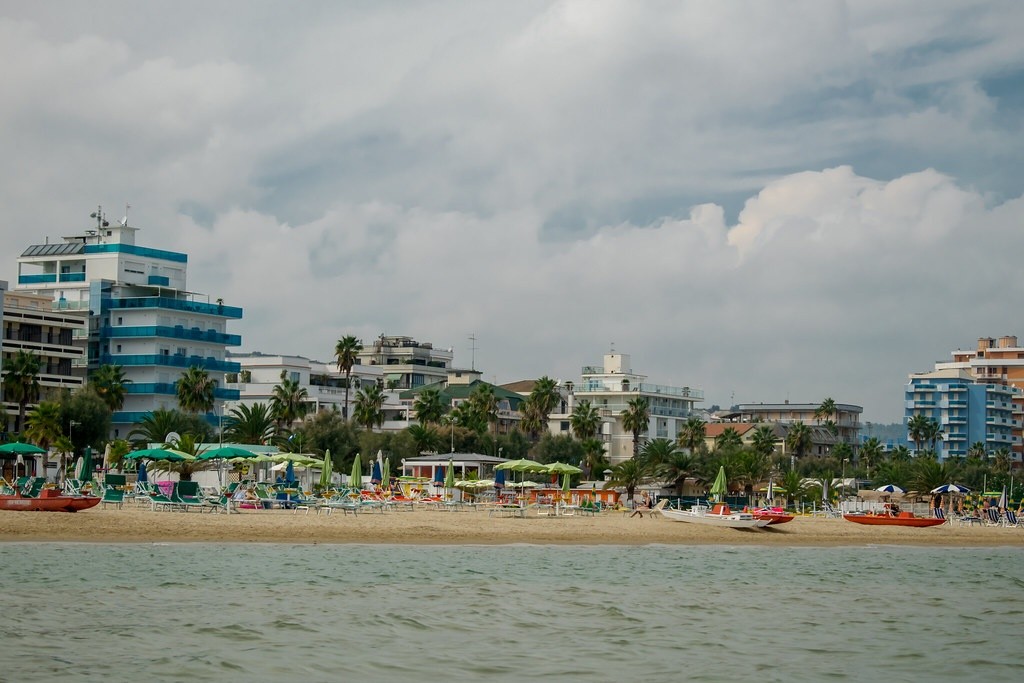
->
[0,474,1024,529]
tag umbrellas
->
[370,450,390,491]
[348,453,361,493]
[103,443,112,472]
[760,483,787,495]
[999,486,1024,527]
[76,446,92,491]
[400,460,495,494]
[710,466,727,502]
[123,447,196,497]
[767,478,773,505]
[197,446,258,485]
[222,449,333,501]
[0,441,47,496]
[487,458,584,497]
[965,491,984,513]
[876,484,909,509]
[930,483,972,524]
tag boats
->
[0,486,102,513]
[707,502,794,525]
[843,505,947,527]
[658,504,773,529]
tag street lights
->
[842,458,849,502]
[451,417,459,454]
[218,404,228,447]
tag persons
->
[630,490,652,518]
[234,491,259,502]
[138,461,147,480]
[972,498,996,517]
